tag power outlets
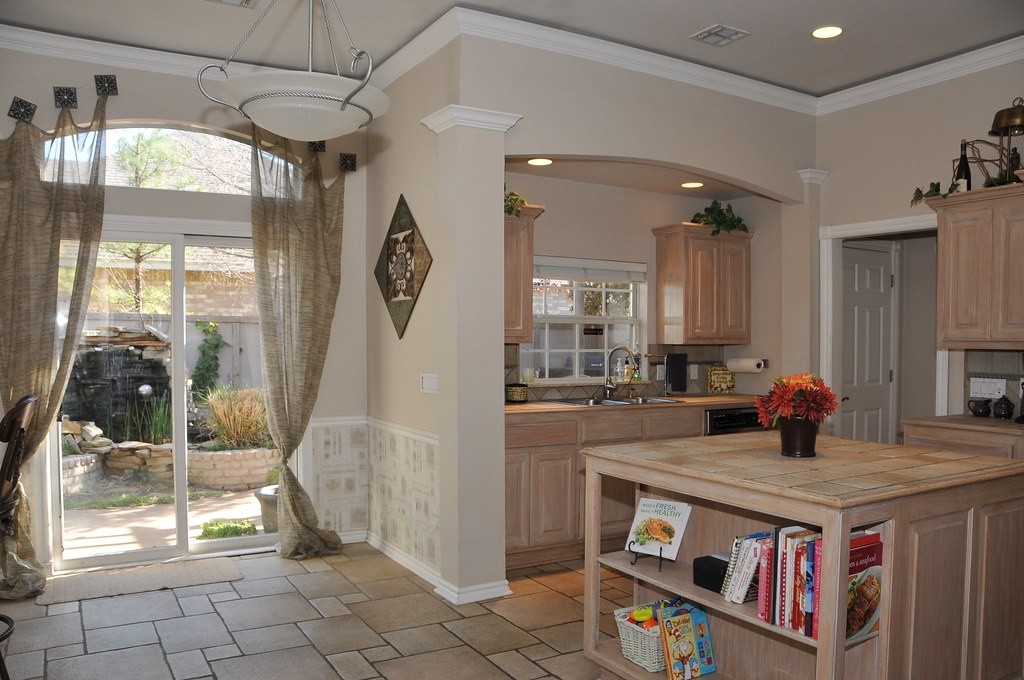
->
[968,378,1008,399]
[657,365,665,380]
[690,364,699,380]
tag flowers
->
[754,372,837,428]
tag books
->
[660,611,715,680]
[707,365,736,394]
[720,524,883,642]
[625,497,691,561]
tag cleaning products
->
[624,355,632,380]
[633,367,641,382]
[614,358,624,382]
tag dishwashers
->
[703,408,779,436]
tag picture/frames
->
[624,497,692,574]
[708,366,736,395]
[373,192,433,340]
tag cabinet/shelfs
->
[504,203,546,344]
[581,432,1024,680]
[938,195,1024,344]
[506,420,580,550]
[908,430,1014,459]
[579,416,702,538]
[652,221,753,346]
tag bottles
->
[993,395,1014,418]
[955,139,972,193]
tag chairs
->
[0,394,38,643]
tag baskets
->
[615,599,667,673]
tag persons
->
[1009,147,1020,174]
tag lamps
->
[197,0,393,143]
[990,98,1024,187]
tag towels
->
[666,353,687,391]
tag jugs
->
[967,398,991,417]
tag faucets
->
[603,345,640,398]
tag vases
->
[253,484,279,534]
[776,413,820,457]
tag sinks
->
[609,397,675,404]
[558,398,627,406]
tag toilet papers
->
[726,358,764,374]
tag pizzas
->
[647,517,676,542]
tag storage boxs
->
[613,599,700,674]
[693,552,730,594]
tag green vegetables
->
[633,520,659,545]
[850,580,860,597]
